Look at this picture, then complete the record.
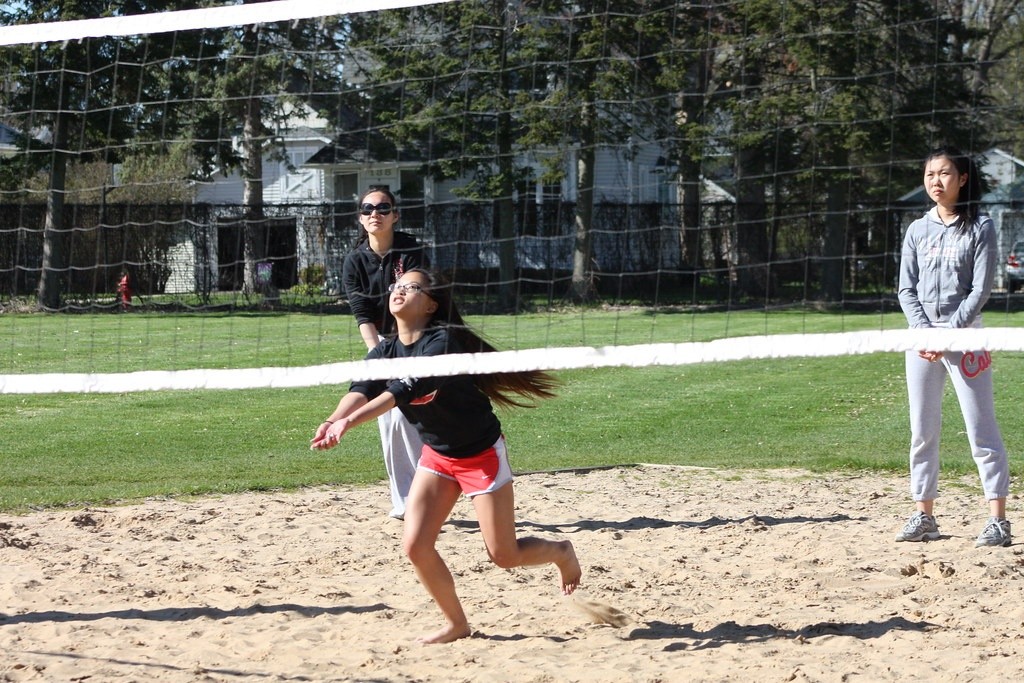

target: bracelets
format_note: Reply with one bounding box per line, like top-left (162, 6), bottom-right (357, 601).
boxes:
top-left (323, 421), bottom-right (334, 424)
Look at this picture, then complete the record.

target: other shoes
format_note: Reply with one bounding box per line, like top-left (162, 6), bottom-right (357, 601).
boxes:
top-left (122, 310), bottom-right (127, 312)
top-left (112, 310), bottom-right (119, 314)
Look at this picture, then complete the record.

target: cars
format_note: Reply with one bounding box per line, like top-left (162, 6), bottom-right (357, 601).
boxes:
top-left (1004, 241), bottom-right (1024, 291)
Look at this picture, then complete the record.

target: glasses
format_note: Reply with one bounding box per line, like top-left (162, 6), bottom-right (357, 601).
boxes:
top-left (388, 284), bottom-right (436, 303)
top-left (359, 202), bottom-right (395, 215)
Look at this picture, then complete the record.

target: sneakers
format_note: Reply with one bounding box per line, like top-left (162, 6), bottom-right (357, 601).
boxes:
top-left (894, 511), bottom-right (941, 542)
top-left (974, 516), bottom-right (1012, 549)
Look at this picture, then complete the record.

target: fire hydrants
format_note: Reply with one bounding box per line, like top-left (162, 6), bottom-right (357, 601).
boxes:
top-left (118, 275), bottom-right (133, 311)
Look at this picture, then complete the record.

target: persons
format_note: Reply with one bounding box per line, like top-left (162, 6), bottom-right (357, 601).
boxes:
top-left (341, 186), bottom-right (431, 521)
top-left (894, 145), bottom-right (1011, 548)
top-left (308, 265), bottom-right (582, 643)
